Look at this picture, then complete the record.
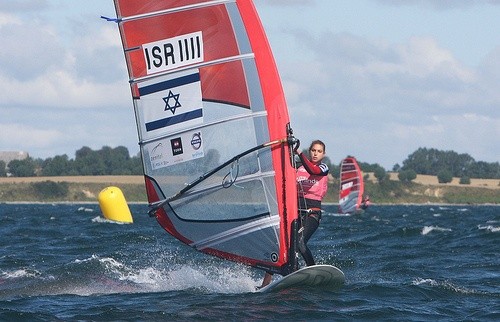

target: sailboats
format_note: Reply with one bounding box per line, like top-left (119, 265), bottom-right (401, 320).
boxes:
top-left (338, 155), bottom-right (366, 218)
top-left (98, 0), bottom-right (344, 295)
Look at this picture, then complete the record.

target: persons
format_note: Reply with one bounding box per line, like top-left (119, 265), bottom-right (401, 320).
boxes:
top-left (360, 194), bottom-right (371, 211)
top-left (287, 140), bottom-right (329, 270)
